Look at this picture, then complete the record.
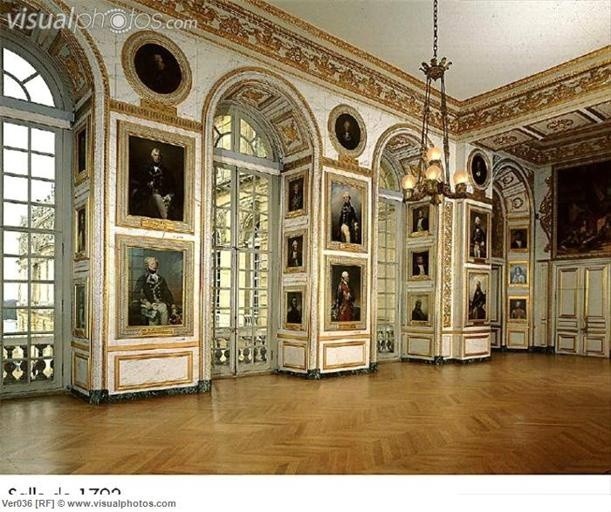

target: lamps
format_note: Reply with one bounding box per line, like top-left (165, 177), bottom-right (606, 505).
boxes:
top-left (399, 1), bottom-right (467, 205)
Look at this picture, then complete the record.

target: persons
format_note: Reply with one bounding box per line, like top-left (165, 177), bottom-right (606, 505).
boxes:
top-left (510, 300), bottom-right (526, 319)
top-left (339, 192), bottom-right (361, 243)
top-left (470, 281), bottom-right (487, 319)
top-left (472, 216), bottom-right (486, 257)
top-left (148, 146), bottom-right (177, 219)
top-left (414, 255), bottom-right (429, 275)
top-left (134, 254), bottom-right (178, 326)
top-left (413, 298), bottom-right (427, 320)
top-left (287, 239), bottom-right (303, 267)
top-left (512, 229), bottom-right (526, 248)
top-left (415, 210), bottom-right (428, 232)
top-left (333, 271), bottom-right (358, 320)
top-left (286, 295), bottom-right (301, 322)
top-left (340, 120), bottom-right (356, 150)
top-left (512, 265), bottom-right (528, 285)
top-left (476, 159), bottom-right (486, 184)
top-left (290, 183), bottom-right (303, 210)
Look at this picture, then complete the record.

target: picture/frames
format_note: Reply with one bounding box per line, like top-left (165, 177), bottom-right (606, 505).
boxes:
top-left (116, 26), bottom-right (195, 340)
top-left (386, 136), bottom-right (529, 323)
top-left (539, 152), bottom-right (610, 259)
top-left (48, 27), bottom-right (93, 108)
top-left (226, 83), bottom-right (370, 331)
top-left (71, 88), bottom-right (100, 351)
